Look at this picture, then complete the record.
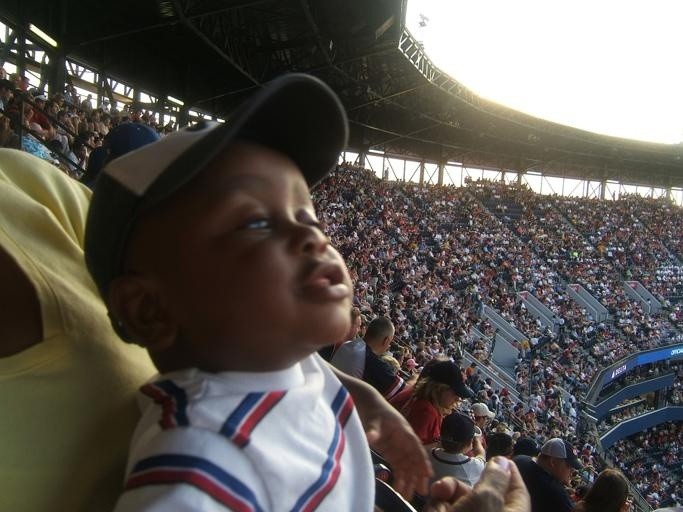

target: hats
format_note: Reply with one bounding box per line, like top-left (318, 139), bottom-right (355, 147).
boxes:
top-left (439, 408), bottom-right (480, 444)
top-left (33, 94), bottom-right (48, 102)
top-left (471, 402), bottom-right (495, 418)
top-left (429, 359), bottom-right (475, 398)
top-left (540, 438), bottom-right (578, 464)
top-left (486, 432), bottom-right (512, 452)
top-left (512, 438), bottom-right (540, 455)
top-left (83, 72), bottom-right (350, 311)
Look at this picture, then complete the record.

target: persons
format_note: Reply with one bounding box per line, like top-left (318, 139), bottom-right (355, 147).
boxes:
top-left (0, 72), bottom-right (683, 511)
